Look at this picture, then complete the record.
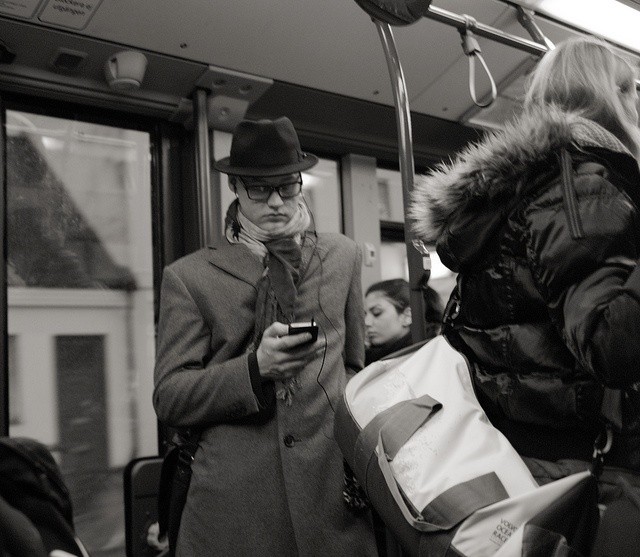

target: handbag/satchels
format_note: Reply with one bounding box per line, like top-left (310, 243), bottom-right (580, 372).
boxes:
top-left (156, 433), bottom-right (194, 556)
top-left (331, 328), bottom-right (600, 557)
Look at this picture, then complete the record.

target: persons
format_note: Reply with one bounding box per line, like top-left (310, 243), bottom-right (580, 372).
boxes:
top-left (362, 279), bottom-right (443, 366)
top-left (405, 35), bottom-right (639, 476)
top-left (153, 114), bottom-right (402, 556)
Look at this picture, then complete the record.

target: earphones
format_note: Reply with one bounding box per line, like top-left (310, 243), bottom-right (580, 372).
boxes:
top-left (229, 178), bottom-right (238, 193)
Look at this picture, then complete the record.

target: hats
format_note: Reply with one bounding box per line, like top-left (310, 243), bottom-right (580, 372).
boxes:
top-left (212, 116), bottom-right (320, 178)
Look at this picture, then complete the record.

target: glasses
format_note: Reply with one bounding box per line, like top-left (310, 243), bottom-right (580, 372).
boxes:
top-left (234, 172), bottom-right (303, 202)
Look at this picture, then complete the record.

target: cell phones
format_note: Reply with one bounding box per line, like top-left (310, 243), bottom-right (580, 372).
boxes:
top-left (288, 321), bottom-right (318, 353)
